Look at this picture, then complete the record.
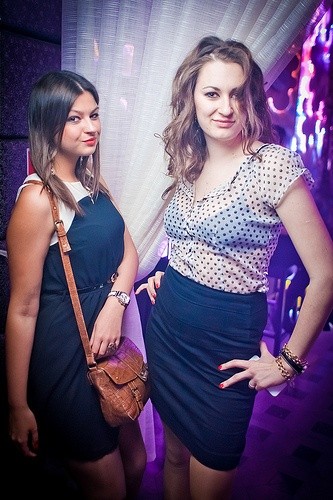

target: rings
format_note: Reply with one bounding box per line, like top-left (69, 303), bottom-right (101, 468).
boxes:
top-left (248, 382), bottom-right (255, 387)
top-left (108, 344), bottom-right (115, 350)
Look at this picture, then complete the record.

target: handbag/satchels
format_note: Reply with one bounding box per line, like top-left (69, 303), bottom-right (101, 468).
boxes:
top-left (86, 336), bottom-right (152, 428)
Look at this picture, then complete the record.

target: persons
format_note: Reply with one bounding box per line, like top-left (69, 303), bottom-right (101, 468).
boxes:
top-left (134, 35), bottom-right (333, 500)
top-left (6, 71), bottom-right (146, 500)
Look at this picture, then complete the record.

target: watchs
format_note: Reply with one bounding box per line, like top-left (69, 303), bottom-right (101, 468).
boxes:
top-left (107, 290), bottom-right (131, 311)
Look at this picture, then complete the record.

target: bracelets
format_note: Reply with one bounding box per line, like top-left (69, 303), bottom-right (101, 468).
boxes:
top-left (275, 345), bottom-right (307, 384)
